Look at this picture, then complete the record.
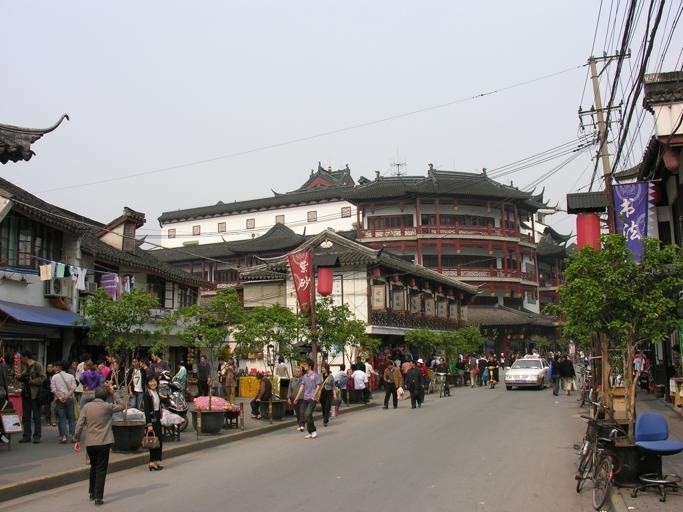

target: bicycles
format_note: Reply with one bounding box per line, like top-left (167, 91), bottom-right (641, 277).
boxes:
top-left (570, 388), bottom-right (625, 508)
top-left (436, 370), bottom-right (452, 397)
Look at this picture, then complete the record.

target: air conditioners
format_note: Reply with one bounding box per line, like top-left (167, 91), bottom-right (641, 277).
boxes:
top-left (45, 279), bottom-right (68, 297)
top-left (81, 282), bottom-right (97, 295)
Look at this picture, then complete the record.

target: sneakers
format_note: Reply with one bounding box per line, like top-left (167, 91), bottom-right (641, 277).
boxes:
top-left (312, 431), bottom-right (318, 437)
top-left (33, 436), bottom-right (40, 443)
top-left (393, 405), bottom-right (397, 409)
top-left (52, 422), bottom-right (57, 426)
top-left (90, 495), bottom-right (95, 500)
top-left (95, 501), bottom-right (104, 505)
top-left (60, 440), bottom-right (67, 443)
top-left (251, 415), bottom-right (257, 417)
top-left (304, 433), bottom-right (311, 438)
top-left (19, 437), bottom-right (30, 443)
top-left (72, 440), bottom-right (78, 443)
top-left (256, 416), bottom-right (262, 419)
top-left (301, 426), bottom-right (305, 430)
top-left (297, 427), bottom-right (301, 431)
top-left (382, 406), bottom-right (387, 408)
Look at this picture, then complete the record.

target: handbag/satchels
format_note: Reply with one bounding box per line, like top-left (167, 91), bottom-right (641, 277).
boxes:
top-left (339, 377), bottom-right (348, 388)
top-left (219, 375), bottom-right (225, 384)
top-left (37, 390), bottom-right (51, 402)
top-left (414, 386), bottom-right (424, 400)
top-left (141, 436), bottom-right (160, 449)
top-left (316, 383), bottom-right (324, 396)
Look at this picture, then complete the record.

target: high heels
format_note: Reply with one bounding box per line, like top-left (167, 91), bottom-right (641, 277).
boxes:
top-left (148, 464), bottom-right (163, 471)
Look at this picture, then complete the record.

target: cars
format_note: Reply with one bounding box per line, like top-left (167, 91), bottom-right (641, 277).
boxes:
top-left (504, 354), bottom-right (554, 390)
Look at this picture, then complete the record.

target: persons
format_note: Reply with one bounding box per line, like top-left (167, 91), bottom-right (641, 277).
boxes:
top-left (172, 355), bottom-right (238, 404)
top-left (382, 357), bottom-right (451, 409)
top-left (548, 351), bottom-right (576, 396)
top-left (0, 353), bottom-right (172, 443)
top-left (250, 356), bottom-right (379, 420)
top-left (294, 358), bottom-right (321, 439)
top-left (457, 353), bottom-right (505, 388)
top-left (71, 382), bottom-right (125, 505)
top-left (143, 373), bottom-right (173, 471)
top-left (286, 366), bottom-right (305, 431)
top-left (672, 344), bottom-right (681, 377)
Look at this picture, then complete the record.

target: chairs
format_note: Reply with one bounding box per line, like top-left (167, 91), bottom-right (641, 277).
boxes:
top-left (630, 413), bottom-right (682, 502)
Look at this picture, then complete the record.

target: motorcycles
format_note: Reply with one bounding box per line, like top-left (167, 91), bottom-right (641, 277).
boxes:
top-left (483, 362), bottom-right (499, 389)
top-left (576, 363), bottom-right (603, 399)
top-left (162, 369), bottom-right (188, 432)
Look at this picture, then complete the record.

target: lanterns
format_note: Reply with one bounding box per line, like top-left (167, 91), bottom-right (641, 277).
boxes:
top-left (423, 280), bottom-right (429, 290)
top-left (448, 289), bottom-right (453, 297)
top-left (437, 285), bottom-right (442, 293)
top-left (459, 293), bottom-right (463, 300)
top-left (409, 278), bottom-right (415, 287)
top-left (392, 273), bottom-right (398, 282)
top-left (576, 212), bottom-right (601, 250)
top-left (372, 268), bottom-right (380, 279)
top-left (317, 268), bottom-right (333, 297)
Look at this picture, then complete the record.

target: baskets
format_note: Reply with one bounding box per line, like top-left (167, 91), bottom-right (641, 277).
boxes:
top-left (587, 422), bottom-right (611, 441)
top-left (436, 376), bottom-right (446, 382)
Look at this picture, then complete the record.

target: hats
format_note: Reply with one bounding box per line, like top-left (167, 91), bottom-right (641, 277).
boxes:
top-left (417, 359), bottom-right (425, 365)
